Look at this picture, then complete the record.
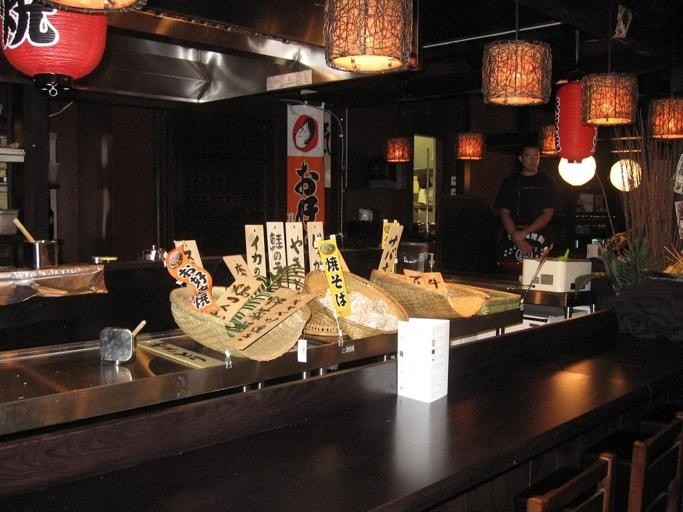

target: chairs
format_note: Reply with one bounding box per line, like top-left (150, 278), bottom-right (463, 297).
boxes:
top-left (512, 400), bottom-right (681, 509)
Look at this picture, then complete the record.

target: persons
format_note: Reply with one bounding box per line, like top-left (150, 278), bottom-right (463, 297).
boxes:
top-left (494, 141), bottom-right (555, 263)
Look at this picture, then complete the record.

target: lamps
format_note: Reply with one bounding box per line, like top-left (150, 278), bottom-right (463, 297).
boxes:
top-left (650, 83), bottom-right (683, 140)
top-left (581, 28), bottom-right (638, 128)
top-left (536, 121), bottom-right (557, 156)
top-left (384, 136), bottom-right (410, 165)
top-left (482, 2), bottom-right (553, 107)
top-left (456, 132), bottom-right (486, 161)
top-left (322, 2), bottom-right (412, 75)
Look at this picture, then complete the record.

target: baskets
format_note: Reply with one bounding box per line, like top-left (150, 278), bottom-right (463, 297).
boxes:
top-left (448, 281), bottom-right (522, 315)
top-left (170, 269), bottom-right (521, 362)
top-left (302, 271), bottom-right (409, 340)
